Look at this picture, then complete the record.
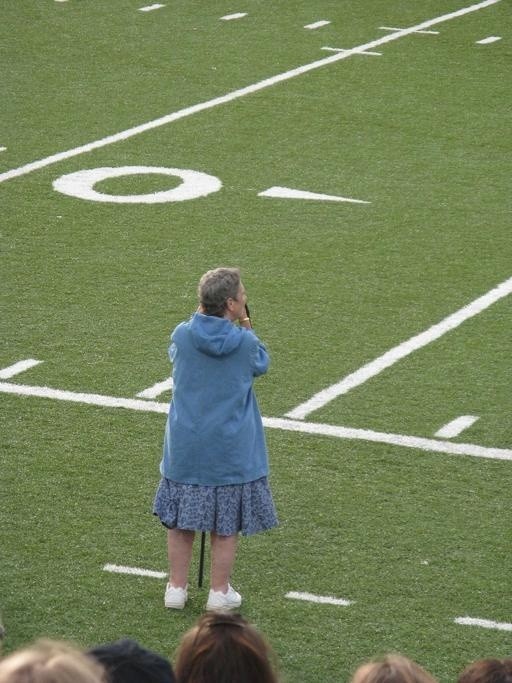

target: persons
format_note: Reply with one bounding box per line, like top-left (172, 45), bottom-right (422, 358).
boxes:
top-left (172, 611), bottom-right (280, 682)
top-left (86, 639), bottom-right (174, 682)
top-left (353, 652), bottom-right (437, 682)
top-left (455, 659), bottom-right (512, 683)
top-left (152, 268), bottom-right (276, 615)
top-left (0, 634), bottom-right (109, 682)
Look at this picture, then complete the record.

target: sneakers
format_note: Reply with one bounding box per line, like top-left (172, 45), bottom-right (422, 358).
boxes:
top-left (163, 583), bottom-right (190, 610)
top-left (207, 583), bottom-right (243, 614)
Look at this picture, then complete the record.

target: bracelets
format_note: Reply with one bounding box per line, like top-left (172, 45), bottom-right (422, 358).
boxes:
top-left (239, 316), bottom-right (250, 324)
